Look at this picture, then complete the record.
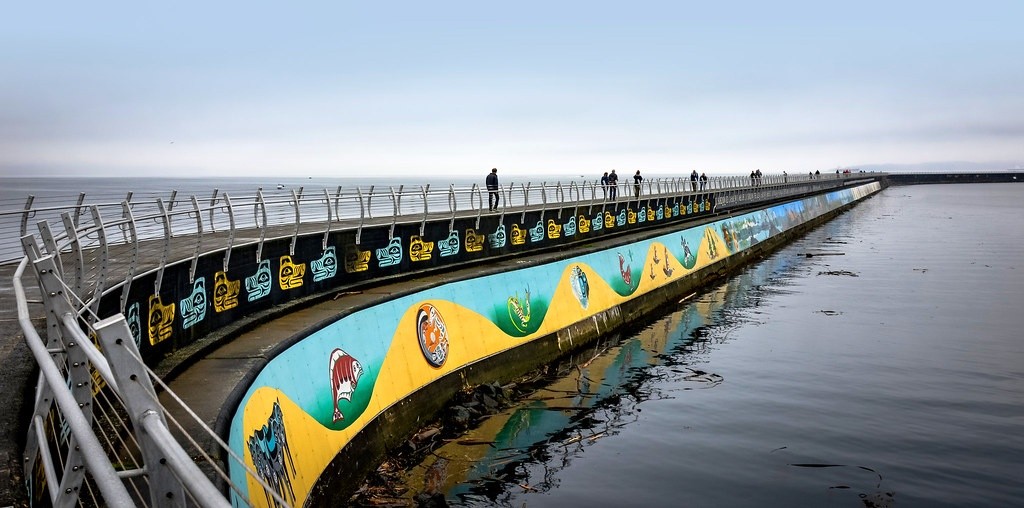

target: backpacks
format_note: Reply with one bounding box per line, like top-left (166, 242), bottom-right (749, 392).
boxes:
top-left (608, 175), bottom-right (617, 186)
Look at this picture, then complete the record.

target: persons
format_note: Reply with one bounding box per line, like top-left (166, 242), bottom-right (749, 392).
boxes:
top-left (633, 170), bottom-right (642, 199)
top-left (783, 171), bottom-right (787, 183)
top-left (750, 169), bottom-right (762, 187)
top-left (836, 170), bottom-right (865, 178)
top-left (601, 172), bottom-right (609, 200)
top-left (609, 170), bottom-right (618, 201)
top-left (809, 172), bottom-right (812, 179)
top-left (691, 170), bottom-right (698, 192)
top-left (700, 173), bottom-right (707, 192)
top-left (815, 170), bottom-right (820, 174)
top-left (486, 168), bottom-right (499, 212)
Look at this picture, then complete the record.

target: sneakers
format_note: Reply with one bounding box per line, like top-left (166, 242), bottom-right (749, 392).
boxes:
top-left (489, 209), bottom-right (497, 212)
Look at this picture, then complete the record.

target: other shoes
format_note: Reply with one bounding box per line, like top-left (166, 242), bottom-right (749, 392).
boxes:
top-left (610, 199), bottom-right (611, 200)
top-left (613, 199), bottom-right (616, 201)
